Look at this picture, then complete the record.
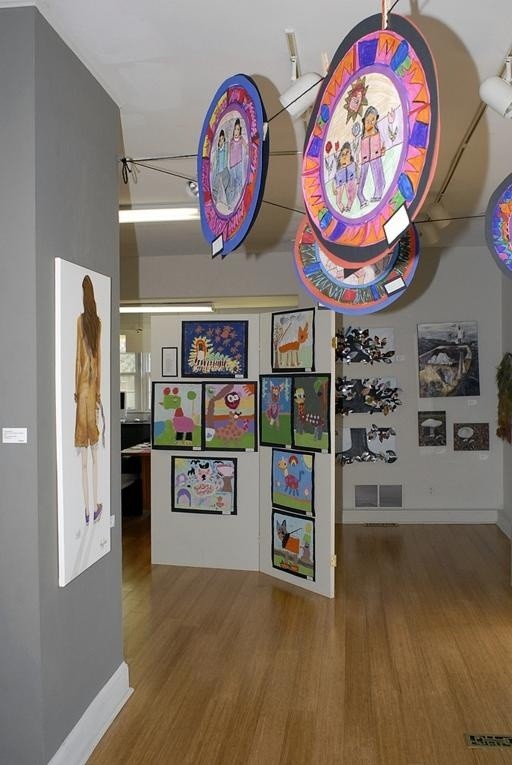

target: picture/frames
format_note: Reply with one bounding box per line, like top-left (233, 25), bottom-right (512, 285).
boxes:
top-left (162, 347), bottom-right (178, 377)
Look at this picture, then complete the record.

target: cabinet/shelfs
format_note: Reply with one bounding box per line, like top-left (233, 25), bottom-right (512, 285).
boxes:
top-left (121, 441), bottom-right (151, 519)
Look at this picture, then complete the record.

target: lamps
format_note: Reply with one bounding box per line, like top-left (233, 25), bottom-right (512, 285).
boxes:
top-left (419, 201), bottom-right (450, 245)
top-left (478, 55), bottom-right (512, 118)
top-left (185, 175), bottom-right (199, 198)
top-left (119, 301), bottom-right (216, 314)
top-left (118, 203), bottom-right (201, 224)
top-left (280, 56), bottom-right (323, 118)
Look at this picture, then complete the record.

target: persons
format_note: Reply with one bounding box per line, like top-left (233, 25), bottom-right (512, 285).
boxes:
top-left (71, 272), bottom-right (109, 531)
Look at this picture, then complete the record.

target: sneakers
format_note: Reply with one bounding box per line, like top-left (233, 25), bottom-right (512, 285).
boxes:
top-left (95, 504), bottom-right (102, 521)
top-left (85, 510), bottom-right (89, 524)
